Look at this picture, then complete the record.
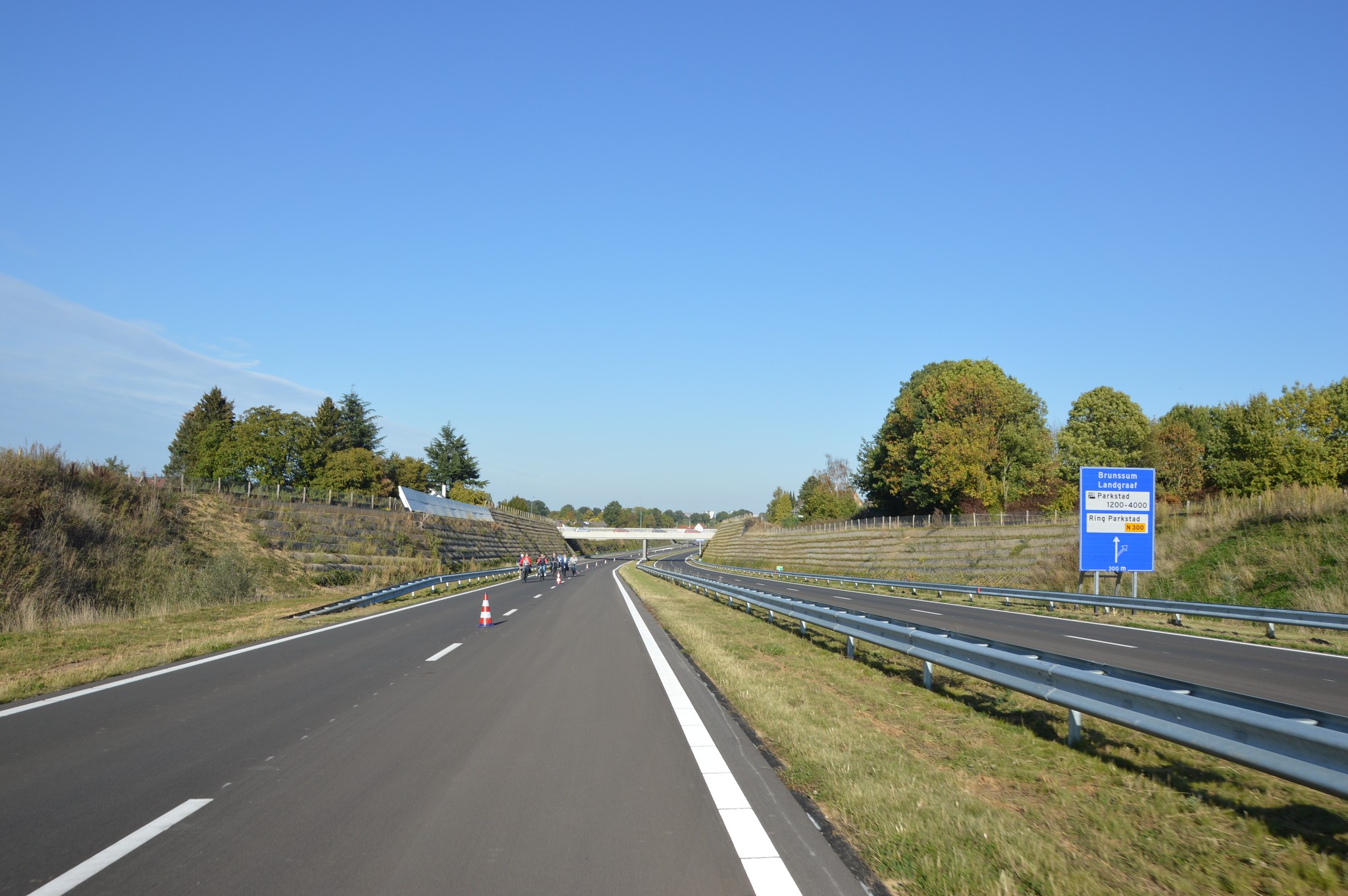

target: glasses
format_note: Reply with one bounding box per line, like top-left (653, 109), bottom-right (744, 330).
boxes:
top-left (541, 556), bottom-right (543, 557)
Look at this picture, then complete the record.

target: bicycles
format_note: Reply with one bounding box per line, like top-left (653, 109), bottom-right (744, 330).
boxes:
top-left (534, 561), bottom-right (577, 581)
top-left (517, 563), bottom-right (530, 583)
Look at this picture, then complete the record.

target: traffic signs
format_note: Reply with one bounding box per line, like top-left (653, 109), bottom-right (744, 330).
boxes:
top-left (1078, 466), bottom-right (1156, 572)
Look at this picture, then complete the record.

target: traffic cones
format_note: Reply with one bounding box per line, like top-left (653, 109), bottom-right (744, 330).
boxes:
top-left (638, 553), bottom-right (640, 556)
top-left (595, 562), bottom-right (598, 567)
top-left (586, 564), bottom-right (589, 570)
top-left (613, 558), bottom-right (616, 562)
top-left (604, 560), bottom-right (607, 564)
top-left (630, 555), bottom-right (632, 558)
top-left (555, 571), bottom-right (563, 585)
top-left (475, 592), bottom-right (496, 628)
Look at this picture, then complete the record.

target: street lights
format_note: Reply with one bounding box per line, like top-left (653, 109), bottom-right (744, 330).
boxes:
top-left (674, 514), bottom-right (678, 528)
top-left (531, 497), bottom-right (535, 518)
top-left (689, 513), bottom-right (693, 529)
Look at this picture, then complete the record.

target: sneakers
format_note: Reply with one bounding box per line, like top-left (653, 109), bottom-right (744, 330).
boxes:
top-left (519, 576), bottom-right (521, 578)
top-left (536, 573), bottom-right (538, 577)
top-left (540, 573), bottom-right (542, 577)
top-left (538, 573), bottom-right (540, 577)
top-left (544, 573), bottom-right (546, 576)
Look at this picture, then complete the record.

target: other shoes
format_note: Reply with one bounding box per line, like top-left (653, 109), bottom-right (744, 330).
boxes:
top-left (526, 573), bottom-right (528, 577)
top-left (554, 571), bottom-right (556, 573)
top-left (551, 571), bottom-right (553, 573)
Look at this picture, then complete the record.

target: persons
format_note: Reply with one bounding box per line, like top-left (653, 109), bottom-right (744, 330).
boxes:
top-left (517, 553), bottom-right (579, 578)
